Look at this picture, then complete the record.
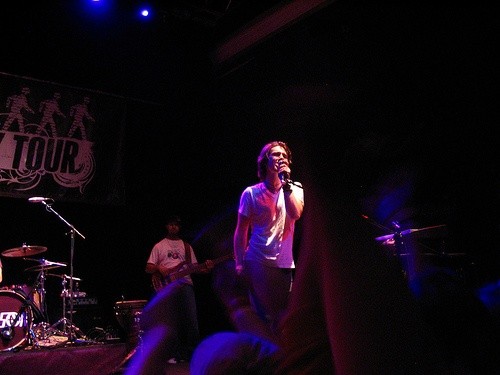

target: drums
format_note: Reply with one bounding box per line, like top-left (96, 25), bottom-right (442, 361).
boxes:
top-left (0, 289), bottom-right (33, 352)
top-left (115, 300), bottom-right (148, 345)
top-left (1, 286), bottom-right (41, 318)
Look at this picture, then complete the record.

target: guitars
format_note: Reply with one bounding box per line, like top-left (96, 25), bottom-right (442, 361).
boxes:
top-left (152, 253), bottom-right (233, 293)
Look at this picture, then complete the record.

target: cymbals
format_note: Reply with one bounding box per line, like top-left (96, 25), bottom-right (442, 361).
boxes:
top-left (1, 245), bottom-right (47, 257)
top-left (25, 262), bottom-right (68, 272)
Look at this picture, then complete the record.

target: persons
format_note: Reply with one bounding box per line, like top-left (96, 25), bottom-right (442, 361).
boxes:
top-left (190, 268), bottom-right (500, 375)
top-left (234, 140), bottom-right (304, 336)
top-left (146, 212), bottom-right (199, 363)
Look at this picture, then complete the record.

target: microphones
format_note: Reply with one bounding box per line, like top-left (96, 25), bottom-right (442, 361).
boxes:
top-left (277, 160), bottom-right (286, 182)
top-left (28, 197), bottom-right (51, 203)
top-left (2, 331), bottom-right (11, 339)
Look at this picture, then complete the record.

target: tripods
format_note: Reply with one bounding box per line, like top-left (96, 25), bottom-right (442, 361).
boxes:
top-left (46, 277), bottom-right (87, 341)
